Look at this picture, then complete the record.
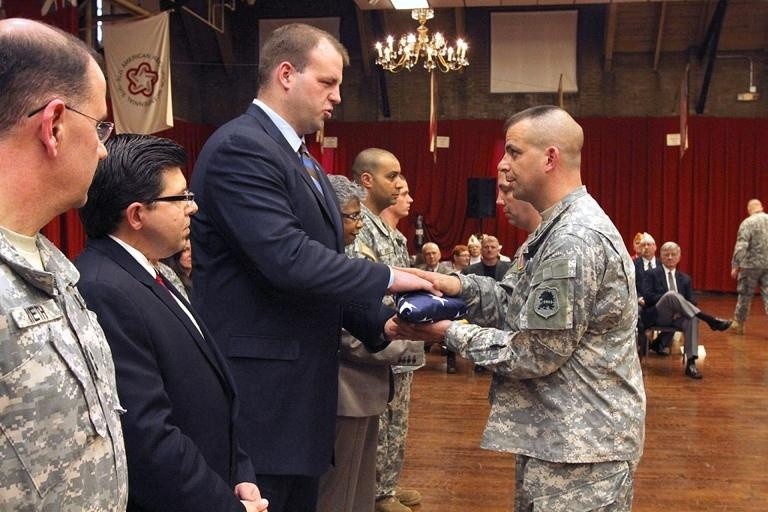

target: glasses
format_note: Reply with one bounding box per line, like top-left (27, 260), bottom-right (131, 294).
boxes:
top-left (455, 253), bottom-right (471, 258)
top-left (28, 100), bottom-right (115, 145)
top-left (147, 190), bottom-right (196, 205)
top-left (341, 212), bottom-right (369, 225)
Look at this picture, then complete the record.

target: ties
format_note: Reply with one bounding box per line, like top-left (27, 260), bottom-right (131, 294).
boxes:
top-left (152, 267), bottom-right (171, 295)
top-left (647, 260), bottom-right (654, 272)
top-left (668, 270), bottom-right (678, 294)
top-left (296, 142), bottom-right (326, 202)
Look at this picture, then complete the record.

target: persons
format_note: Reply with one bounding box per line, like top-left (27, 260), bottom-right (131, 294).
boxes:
top-left (633, 235), bottom-right (675, 355)
top-left (464, 236), bottom-right (511, 280)
top-left (479, 233), bottom-right (490, 242)
top-left (321, 168), bottom-right (400, 512)
top-left (627, 231), bottom-right (644, 261)
top-left (441, 245), bottom-right (468, 276)
top-left (390, 106), bottom-right (647, 512)
top-left (1, 16), bottom-right (129, 512)
top-left (72, 132), bottom-right (270, 512)
top-left (456, 155), bottom-right (543, 512)
top-left (190, 22), bottom-right (441, 512)
top-left (379, 174), bottom-right (429, 512)
top-left (728, 199), bottom-right (768, 331)
top-left (639, 240), bottom-right (732, 381)
top-left (466, 235), bottom-right (482, 266)
top-left (348, 141), bottom-right (401, 512)
top-left (411, 240), bottom-right (452, 275)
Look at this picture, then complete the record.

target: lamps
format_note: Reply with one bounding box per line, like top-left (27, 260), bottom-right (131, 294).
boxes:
top-left (374, 9), bottom-right (469, 73)
top-left (390, 0), bottom-right (430, 9)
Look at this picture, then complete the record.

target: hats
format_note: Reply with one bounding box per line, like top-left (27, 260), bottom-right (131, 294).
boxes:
top-left (468, 235), bottom-right (480, 247)
top-left (640, 233), bottom-right (655, 244)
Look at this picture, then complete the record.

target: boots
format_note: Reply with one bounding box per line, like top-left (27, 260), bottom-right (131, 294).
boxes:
top-left (392, 484), bottom-right (423, 507)
top-left (375, 493), bottom-right (409, 512)
top-left (728, 318), bottom-right (746, 337)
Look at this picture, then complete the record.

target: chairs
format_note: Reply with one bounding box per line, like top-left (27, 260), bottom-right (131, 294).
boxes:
top-left (645, 325), bottom-right (686, 369)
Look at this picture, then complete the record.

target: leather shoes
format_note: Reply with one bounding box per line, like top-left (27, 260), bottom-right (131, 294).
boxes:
top-left (653, 338), bottom-right (670, 356)
top-left (684, 362), bottom-right (704, 382)
top-left (709, 318), bottom-right (735, 333)
